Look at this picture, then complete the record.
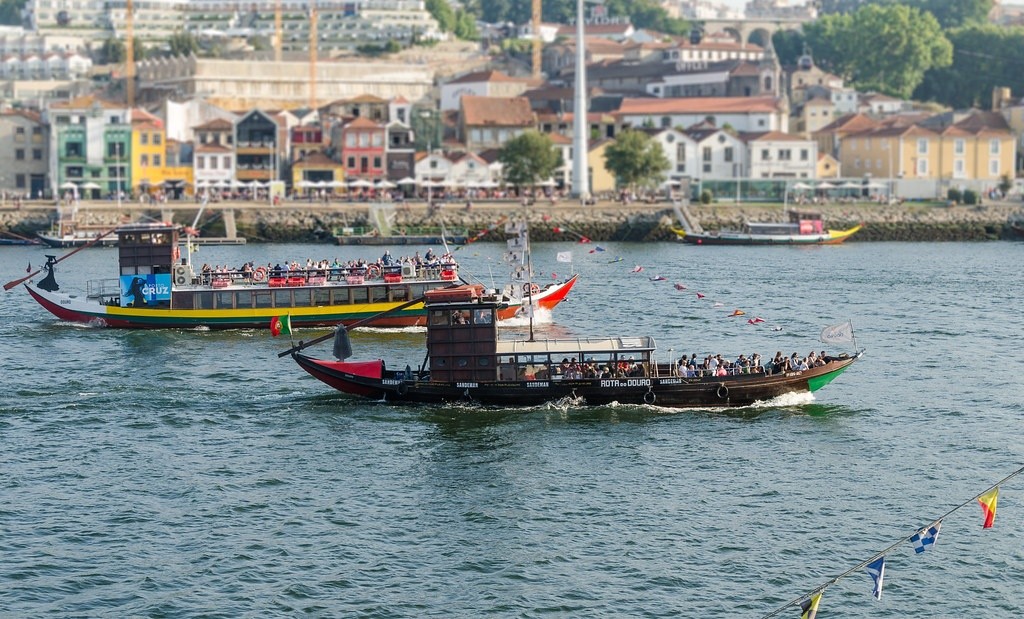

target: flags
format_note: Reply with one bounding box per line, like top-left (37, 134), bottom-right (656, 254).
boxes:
top-left (440, 211), bottom-right (783, 333)
top-left (977, 487), bottom-right (998, 528)
top-left (908, 521), bottom-right (943, 553)
top-left (864, 557), bottom-right (886, 600)
top-left (798, 591), bottom-right (822, 619)
top-left (821, 324), bottom-right (853, 343)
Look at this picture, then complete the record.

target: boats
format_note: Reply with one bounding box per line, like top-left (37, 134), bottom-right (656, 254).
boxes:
top-left (35, 192), bottom-right (248, 248)
top-left (23, 180), bottom-right (580, 330)
top-left (669, 191), bottom-right (863, 246)
top-left (280, 227), bottom-right (868, 407)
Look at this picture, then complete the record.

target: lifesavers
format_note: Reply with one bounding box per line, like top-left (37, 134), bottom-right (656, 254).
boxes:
top-left (715, 386), bottom-right (730, 399)
top-left (523, 282), bottom-right (530, 293)
top-left (252, 267), bottom-right (267, 283)
top-left (397, 382), bottom-right (409, 395)
top-left (531, 284), bottom-right (541, 294)
top-left (643, 391), bottom-right (658, 405)
top-left (365, 263), bottom-right (381, 279)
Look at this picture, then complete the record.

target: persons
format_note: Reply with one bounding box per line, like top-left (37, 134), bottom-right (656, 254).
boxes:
top-left (36, 257), bottom-right (60, 292)
top-left (477, 310), bottom-right (486, 324)
top-left (406, 350), bottom-right (831, 379)
top-left (201, 247), bottom-right (455, 283)
top-left (124, 277), bottom-right (146, 307)
top-left (0, 187), bottom-right (904, 238)
top-left (452, 311), bottom-right (468, 325)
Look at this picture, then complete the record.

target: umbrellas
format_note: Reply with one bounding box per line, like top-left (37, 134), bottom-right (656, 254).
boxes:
top-left (53, 177), bottom-right (886, 190)
